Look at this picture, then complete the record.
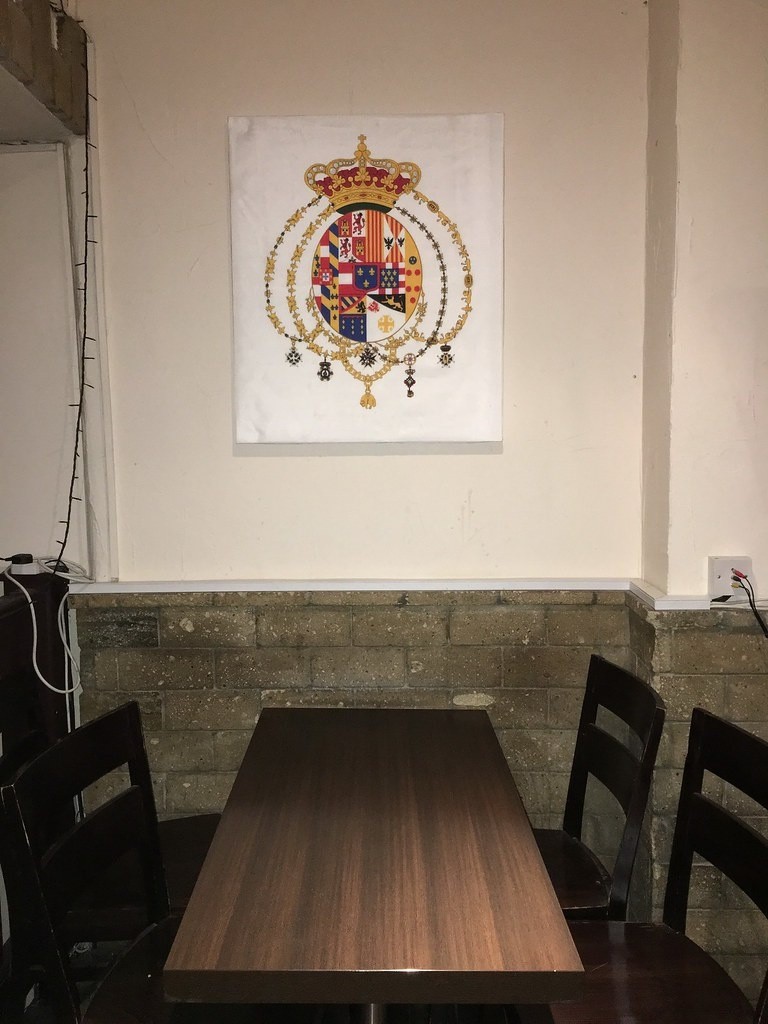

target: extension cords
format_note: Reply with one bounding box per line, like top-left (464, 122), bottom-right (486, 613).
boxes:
top-left (11, 561), bottom-right (47, 574)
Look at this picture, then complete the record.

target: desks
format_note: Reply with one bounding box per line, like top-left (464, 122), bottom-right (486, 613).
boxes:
top-left (161, 708), bottom-right (586, 1024)
top-left (0, 569), bottom-right (75, 975)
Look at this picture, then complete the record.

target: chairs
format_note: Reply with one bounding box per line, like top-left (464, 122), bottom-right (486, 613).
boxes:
top-left (507, 705), bottom-right (768, 1024)
top-left (0, 700), bottom-right (351, 1024)
top-left (532, 652), bottom-right (668, 920)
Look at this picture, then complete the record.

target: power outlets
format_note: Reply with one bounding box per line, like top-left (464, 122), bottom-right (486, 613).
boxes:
top-left (707, 556), bottom-right (753, 602)
